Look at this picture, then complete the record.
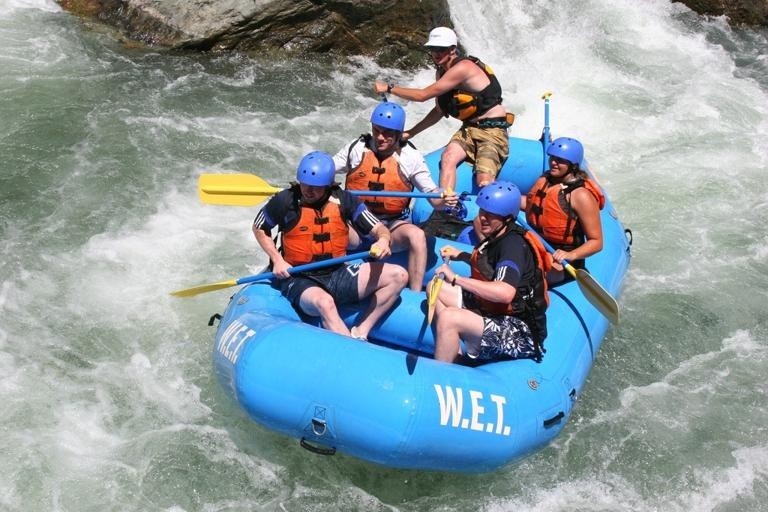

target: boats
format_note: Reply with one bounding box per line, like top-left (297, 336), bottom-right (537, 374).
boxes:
top-left (204, 130), bottom-right (638, 476)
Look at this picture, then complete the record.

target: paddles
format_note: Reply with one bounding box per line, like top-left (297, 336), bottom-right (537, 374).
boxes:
top-left (428, 255), bottom-right (450, 325)
top-left (515, 216), bottom-right (619, 327)
top-left (199, 173), bottom-right (453, 206)
top-left (172, 248), bottom-right (379, 299)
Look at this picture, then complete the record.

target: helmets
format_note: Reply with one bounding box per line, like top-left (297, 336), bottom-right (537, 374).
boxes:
top-left (296, 151), bottom-right (335, 187)
top-left (546, 137), bottom-right (584, 167)
top-left (474, 181), bottom-right (521, 220)
top-left (370, 102), bottom-right (405, 132)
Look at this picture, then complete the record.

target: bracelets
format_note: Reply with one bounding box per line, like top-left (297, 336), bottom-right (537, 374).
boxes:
top-left (452, 274), bottom-right (459, 286)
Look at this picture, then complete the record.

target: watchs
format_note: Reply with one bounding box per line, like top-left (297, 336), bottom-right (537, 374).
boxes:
top-left (387, 83), bottom-right (394, 93)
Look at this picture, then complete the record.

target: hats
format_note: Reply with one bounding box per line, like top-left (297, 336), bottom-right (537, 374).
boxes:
top-left (423, 26), bottom-right (457, 48)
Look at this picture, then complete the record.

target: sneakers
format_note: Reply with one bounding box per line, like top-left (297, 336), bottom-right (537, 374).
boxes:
top-left (349, 326), bottom-right (368, 342)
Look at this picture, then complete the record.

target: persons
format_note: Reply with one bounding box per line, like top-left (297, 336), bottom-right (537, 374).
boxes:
top-left (332, 102), bottom-right (459, 291)
top-left (474, 137), bottom-right (605, 290)
top-left (374, 27), bottom-right (509, 194)
top-left (426, 181), bottom-right (550, 364)
top-left (252, 150), bottom-right (408, 341)
